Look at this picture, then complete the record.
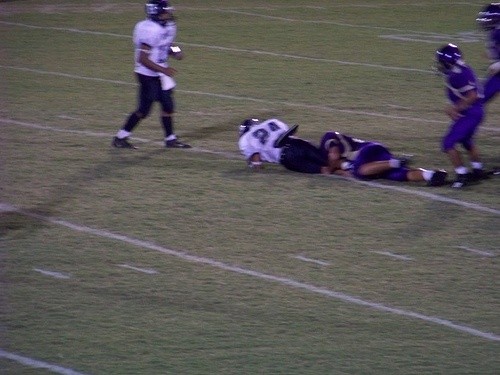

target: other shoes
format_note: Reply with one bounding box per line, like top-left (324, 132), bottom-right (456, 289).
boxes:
top-left (490, 167), bottom-right (500, 175)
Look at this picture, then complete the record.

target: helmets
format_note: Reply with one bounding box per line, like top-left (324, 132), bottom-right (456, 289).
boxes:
top-left (144, 0), bottom-right (176, 21)
top-left (435, 44), bottom-right (462, 74)
top-left (239, 118), bottom-right (264, 137)
top-left (476, 3), bottom-right (500, 30)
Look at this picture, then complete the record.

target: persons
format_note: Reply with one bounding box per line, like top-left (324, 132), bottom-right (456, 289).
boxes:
top-left (321, 131), bottom-right (447, 183)
top-left (435, 43), bottom-right (484, 189)
top-left (476, 3), bottom-right (500, 100)
top-left (239, 118), bottom-right (351, 177)
top-left (112, 0), bottom-right (192, 149)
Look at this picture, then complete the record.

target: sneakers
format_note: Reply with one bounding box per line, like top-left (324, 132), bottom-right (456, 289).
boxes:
top-left (469, 169), bottom-right (487, 179)
top-left (430, 169), bottom-right (447, 186)
top-left (113, 137), bottom-right (137, 149)
top-left (452, 174), bottom-right (470, 188)
top-left (167, 141), bottom-right (192, 148)
top-left (394, 158), bottom-right (410, 166)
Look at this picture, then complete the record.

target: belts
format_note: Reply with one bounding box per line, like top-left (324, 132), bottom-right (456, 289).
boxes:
top-left (280, 142), bottom-right (291, 164)
